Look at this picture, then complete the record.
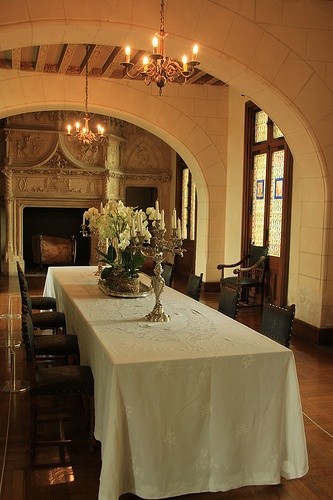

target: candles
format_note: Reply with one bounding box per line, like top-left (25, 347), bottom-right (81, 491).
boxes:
top-left (155, 199), bottom-right (160, 220)
top-left (83, 212), bottom-right (85, 225)
top-left (131, 218), bottom-right (136, 236)
top-left (177, 217), bottom-right (182, 239)
top-left (160, 209), bottom-right (165, 228)
top-left (137, 209), bottom-right (143, 231)
top-left (172, 208), bottom-right (176, 228)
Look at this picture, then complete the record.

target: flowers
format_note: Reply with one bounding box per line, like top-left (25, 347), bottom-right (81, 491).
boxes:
top-left (86, 200), bottom-right (166, 279)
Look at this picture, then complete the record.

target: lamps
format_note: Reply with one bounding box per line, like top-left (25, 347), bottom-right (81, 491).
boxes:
top-left (119, 0), bottom-right (201, 97)
top-left (66, 46), bottom-right (106, 151)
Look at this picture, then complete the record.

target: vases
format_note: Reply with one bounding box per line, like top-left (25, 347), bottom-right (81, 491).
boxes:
top-left (98, 267), bottom-right (151, 299)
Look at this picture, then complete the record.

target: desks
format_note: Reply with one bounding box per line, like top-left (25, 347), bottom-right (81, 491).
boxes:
top-left (40, 266), bottom-right (309, 500)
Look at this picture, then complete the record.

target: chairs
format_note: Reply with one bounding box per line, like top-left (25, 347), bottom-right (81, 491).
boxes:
top-left (216, 279), bottom-right (241, 319)
top-left (14, 261), bottom-right (102, 500)
top-left (217, 242), bottom-right (269, 314)
top-left (260, 301), bottom-right (296, 350)
top-left (161, 261), bottom-right (176, 287)
top-left (186, 270), bottom-right (205, 302)
